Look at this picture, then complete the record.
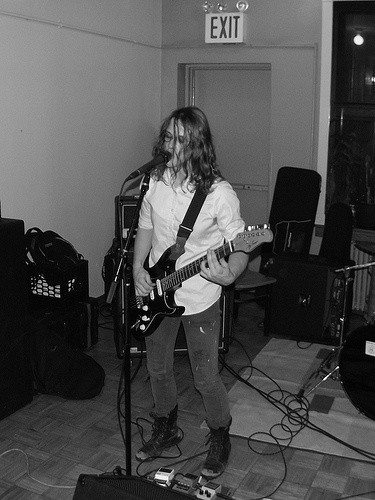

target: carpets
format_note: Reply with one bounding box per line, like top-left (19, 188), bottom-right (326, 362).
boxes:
top-left (201, 338), bottom-right (375, 463)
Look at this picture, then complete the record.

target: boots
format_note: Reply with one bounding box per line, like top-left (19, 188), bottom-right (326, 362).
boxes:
top-left (201, 422), bottom-right (232, 479)
top-left (136, 404), bottom-right (179, 463)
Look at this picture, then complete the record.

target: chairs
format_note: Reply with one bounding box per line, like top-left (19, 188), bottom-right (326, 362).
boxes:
top-left (223, 268), bottom-right (276, 344)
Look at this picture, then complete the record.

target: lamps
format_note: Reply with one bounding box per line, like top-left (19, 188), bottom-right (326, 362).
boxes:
top-left (353, 29), bottom-right (364, 45)
top-left (200, 0), bottom-right (249, 45)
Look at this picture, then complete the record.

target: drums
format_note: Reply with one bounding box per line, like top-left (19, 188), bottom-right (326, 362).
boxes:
top-left (338, 322), bottom-right (375, 422)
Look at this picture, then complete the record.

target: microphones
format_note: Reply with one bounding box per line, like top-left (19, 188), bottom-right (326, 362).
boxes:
top-left (124, 151), bottom-right (172, 183)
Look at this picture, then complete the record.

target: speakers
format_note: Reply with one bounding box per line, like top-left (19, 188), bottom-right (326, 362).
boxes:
top-left (0, 217), bottom-right (35, 421)
top-left (115, 247), bottom-right (232, 358)
top-left (258, 166), bottom-right (356, 343)
top-left (73, 474), bottom-right (201, 500)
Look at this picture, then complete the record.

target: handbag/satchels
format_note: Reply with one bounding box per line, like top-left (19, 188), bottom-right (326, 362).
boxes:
top-left (38, 349), bottom-right (104, 400)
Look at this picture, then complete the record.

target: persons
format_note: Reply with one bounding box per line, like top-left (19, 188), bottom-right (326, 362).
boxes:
top-left (134, 107), bottom-right (251, 481)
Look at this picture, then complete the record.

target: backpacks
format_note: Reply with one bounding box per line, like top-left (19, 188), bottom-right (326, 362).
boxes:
top-left (24, 227), bottom-right (83, 287)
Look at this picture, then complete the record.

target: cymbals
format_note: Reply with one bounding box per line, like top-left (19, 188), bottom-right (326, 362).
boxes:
top-left (353, 239), bottom-right (375, 254)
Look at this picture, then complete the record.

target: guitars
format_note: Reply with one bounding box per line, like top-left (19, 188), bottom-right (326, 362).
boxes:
top-left (125, 219), bottom-right (275, 340)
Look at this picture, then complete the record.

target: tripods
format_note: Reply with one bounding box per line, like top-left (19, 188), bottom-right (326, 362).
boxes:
top-left (297, 261), bottom-right (375, 399)
